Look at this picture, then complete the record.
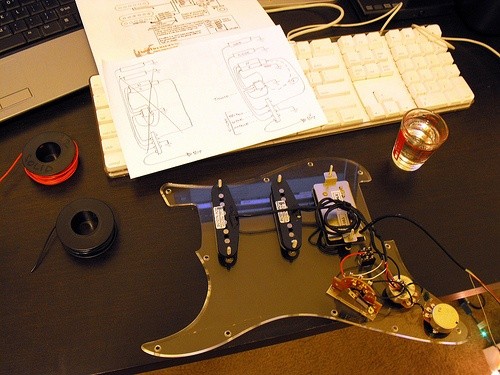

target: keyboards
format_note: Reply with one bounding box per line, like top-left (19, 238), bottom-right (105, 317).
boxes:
top-left (89, 23), bottom-right (476, 178)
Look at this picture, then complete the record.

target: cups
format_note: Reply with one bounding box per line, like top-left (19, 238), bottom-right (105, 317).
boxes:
top-left (392, 108), bottom-right (448, 170)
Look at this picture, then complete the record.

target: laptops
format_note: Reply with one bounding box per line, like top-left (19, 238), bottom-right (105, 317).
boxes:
top-left (1, 0), bottom-right (99, 123)
